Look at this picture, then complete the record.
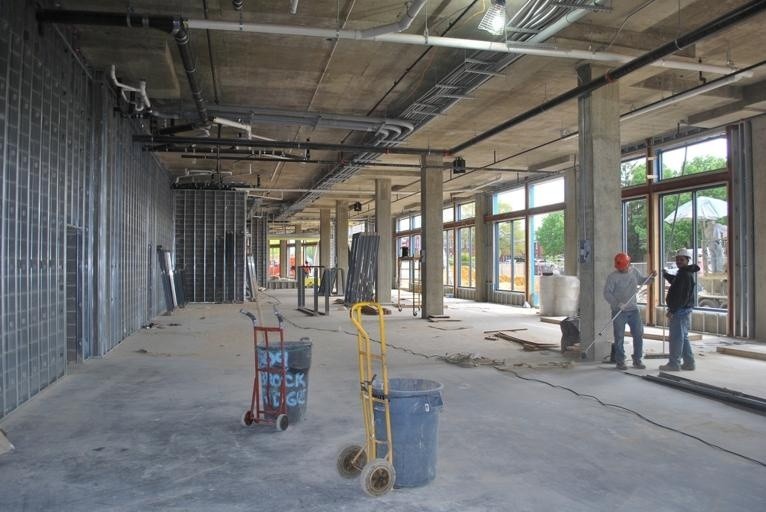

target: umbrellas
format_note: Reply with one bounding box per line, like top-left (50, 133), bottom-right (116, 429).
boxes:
top-left (664, 196), bottom-right (728, 272)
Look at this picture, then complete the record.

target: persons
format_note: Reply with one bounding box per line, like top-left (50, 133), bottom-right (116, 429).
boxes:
top-left (304, 261), bottom-right (311, 279)
top-left (706, 220), bottom-right (723, 274)
top-left (604, 253), bottom-right (657, 370)
top-left (659, 247), bottom-right (700, 370)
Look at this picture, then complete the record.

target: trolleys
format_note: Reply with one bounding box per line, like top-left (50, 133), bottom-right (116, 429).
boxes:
top-left (336, 302), bottom-right (396, 497)
top-left (240, 308), bottom-right (289, 432)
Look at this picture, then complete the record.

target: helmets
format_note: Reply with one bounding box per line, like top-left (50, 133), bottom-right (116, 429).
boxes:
top-left (615, 254), bottom-right (631, 268)
top-left (675, 248), bottom-right (692, 260)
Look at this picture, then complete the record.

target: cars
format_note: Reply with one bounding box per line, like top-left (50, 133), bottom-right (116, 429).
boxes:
top-left (535, 258), bottom-right (558, 275)
top-left (664, 260), bottom-right (713, 299)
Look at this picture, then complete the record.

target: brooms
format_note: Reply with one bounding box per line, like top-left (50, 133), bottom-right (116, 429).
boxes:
top-left (645, 274), bottom-right (672, 359)
top-left (563, 272), bottom-right (654, 363)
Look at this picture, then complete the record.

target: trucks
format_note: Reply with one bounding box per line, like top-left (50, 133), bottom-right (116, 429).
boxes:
top-left (269, 255), bottom-right (295, 279)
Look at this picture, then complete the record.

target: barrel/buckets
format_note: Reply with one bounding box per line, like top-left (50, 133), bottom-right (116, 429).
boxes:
top-left (539, 272), bottom-right (581, 317)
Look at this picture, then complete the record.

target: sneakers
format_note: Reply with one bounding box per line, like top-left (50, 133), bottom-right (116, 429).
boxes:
top-left (616, 360), bottom-right (628, 370)
top-left (659, 362), bottom-right (695, 371)
top-left (633, 360), bottom-right (646, 369)
